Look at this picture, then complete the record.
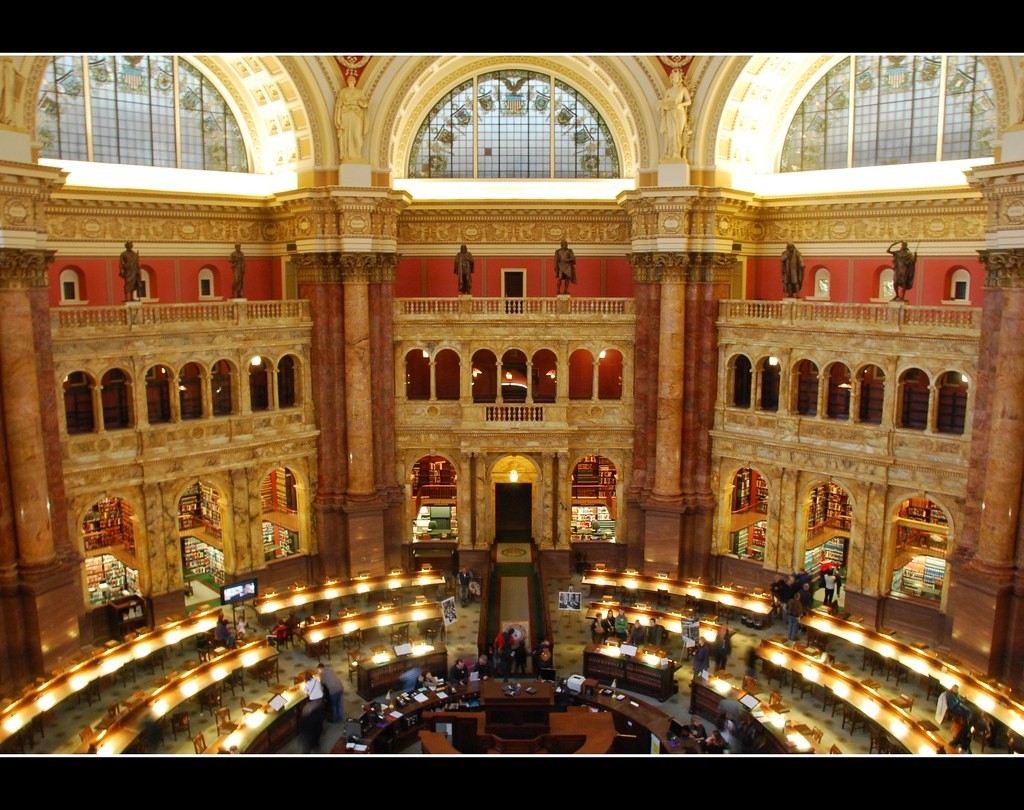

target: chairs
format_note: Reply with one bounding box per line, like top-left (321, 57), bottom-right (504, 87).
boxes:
top-left (443, 576), bottom-right (459, 602)
top-left (596, 563), bottom-right (1024, 754)
top-left (253, 564), bottom-right (438, 683)
top-left (0, 604), bottom-right (317, 754)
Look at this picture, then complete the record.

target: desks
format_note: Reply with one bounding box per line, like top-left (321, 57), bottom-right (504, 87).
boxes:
top-left (291, 601), bottom-right (443, 658)
top-left (479, 680), bottom-right (555, 725)
top-left (0, 608), bottom-right (229, 753)
top-left (417, 730), bottom-right (462, 754)
top-left (585, 601), bottom-right (736, 660)
top-left (356, 643), bottom-right (448, 701)
top-left (555, 683), bottom-right (709, 754)
top-left (756, 640), bottom-right (959, 754)
top-left (688, 675), bottom-right (827, 755)
top-left (582, 568), bottom-right (783, 630)
top-left (583, 643), bottom-right (683, 703)
top-left (549, 712), bottom-right (616, 753)
top-left (202, 673), bottom-right (330, 754)
top-left (72, 638), bottom-right (280, 754)
top-left (254, 569), bottom-right (445, 628)
top-left (799, 609), bottom-right (1024, 737)
top-left (330, 681), bottom-right (480, 756)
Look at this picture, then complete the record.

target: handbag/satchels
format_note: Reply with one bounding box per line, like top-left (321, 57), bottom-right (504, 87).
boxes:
top-left (818, 580), bottom-right (826, 588)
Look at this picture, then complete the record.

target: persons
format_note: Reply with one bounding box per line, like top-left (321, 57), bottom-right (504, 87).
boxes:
top-left (887, 240), bottom-right (917, 301)
top-left (780, 242), bottom-right (805, 298)
top-left (266, 611), bottom-right (294, 646)
top-left (414, 671), bottom-right (438, 690)
top-left (591, 607), bottom-right (663, 650)
top-left (235, 617), bottom-right (248, 641)
top-left (492, 628), bottom-right (535, 683)
top-left (944, 685), bottom-right (975, 754)
top-left (770, 567), bottom-right (837, 641)
top-left (118, 240), bottom-right (141, 301)
top-left (554, 240), bottom-right (575, 295)
top-left (683, 714), bottom-right (727, 755)
top-left (457, 567), bottom-right (473, 608)
top-left (213, 614), bottom-right (236, 648)
top-left (449, 658), bottom-right (470, 685)
top-left (303, 664), bottom-right (344, 726)
top-left (692, 638), bottom-right (710, 678)
top-left (228, 243), bottom-right (246, 298)
top-left (453, 244), bottom-right (475, 294)
top-left (714, 625), bottom-right (733, 675)
top-left (471, 655), bottom-right (492, 681)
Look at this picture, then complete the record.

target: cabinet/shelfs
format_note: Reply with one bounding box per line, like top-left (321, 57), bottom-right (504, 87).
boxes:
top-left (109, 595), bottom-right (150, 640)
top-left (732, 468), bottom-right (768, 514)
top-left (572, 455), bottom-right (617, 498)
top-left (177, 479), bottom-right (222, 541)
top-left (81, 495), bottom-right (135, 555)
top-left (260, 467), bottom-right (297, 514)
top-left (411, 455), bottom-right (457, 498)
top-left (85, 556), bottom-right (140, 594)
top-left (892, 497), bottom-right (948, 556)
top-left (806, 481), bottom-right (853, 540)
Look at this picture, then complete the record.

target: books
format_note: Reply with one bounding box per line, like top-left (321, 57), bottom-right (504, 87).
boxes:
top-left (78, 470), bottom-right (294, 605)
top-left (737, 468), bottom-right (847, 568)
top-left (572, 456), bottom-right (615, 497)
top-left (412, 463), bottom-right (456, 485)
top-left (892, 501), bottom-right (948, 600)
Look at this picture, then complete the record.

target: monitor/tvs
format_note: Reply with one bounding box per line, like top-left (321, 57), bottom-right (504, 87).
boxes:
top-left (220, 577), bottom-right (257, 605)
